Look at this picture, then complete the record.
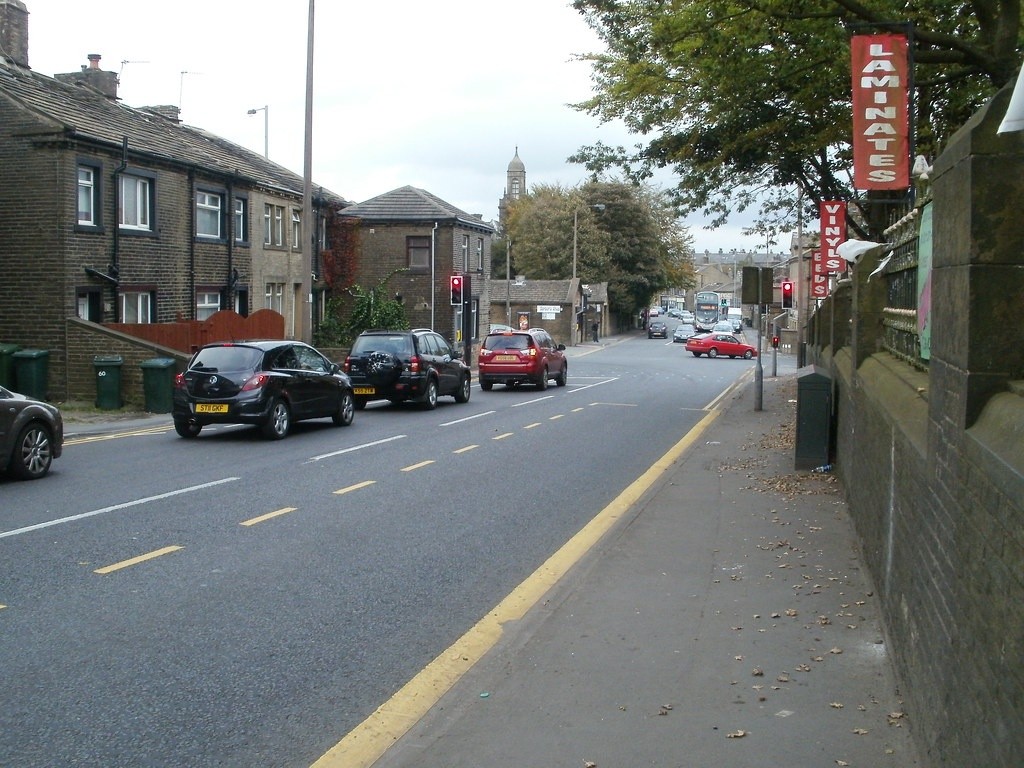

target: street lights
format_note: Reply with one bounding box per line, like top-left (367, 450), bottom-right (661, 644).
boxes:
top-left (572, 203), bottom-right (604, 277)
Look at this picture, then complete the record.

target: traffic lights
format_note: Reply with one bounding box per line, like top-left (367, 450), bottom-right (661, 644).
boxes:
top-left (450, 275), bottom-right (463, 306)
top-left (772, 336), bottom-right (779, 347)
top-left (782, 281), bottom-right (792, 309)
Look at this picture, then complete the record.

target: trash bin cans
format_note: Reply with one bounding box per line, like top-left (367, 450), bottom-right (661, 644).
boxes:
top-left (12, 349), bottom-right (53, 402)
top-left (92, 355), bottom-right (125, 409)
top-left (0, 343), bottom-right (22, 391)
top-left (793, 364), bottom-right (836, 471)
top-left (139, 358), bottom-right (177, 413)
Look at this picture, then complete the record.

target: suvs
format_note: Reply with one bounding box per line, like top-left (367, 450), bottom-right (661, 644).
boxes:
top-left (343, 327), bottom-right (473, 410)
top-left (477, 327), bottom-right (567, 393)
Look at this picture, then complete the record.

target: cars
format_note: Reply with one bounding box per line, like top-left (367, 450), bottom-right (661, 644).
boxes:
top-left (648, 321), bottom-right (668, 339)
top-left (171, 337), bottom-right (354, 440)
top-left (648, 305), bottom-right (694, 323)
top-left (710, 307), bottom-right (744, 339)
top-left (685, 332), bottom-right (757, 360)
top-left (1, 380), bottom-right (65, 478)
top-left (672, 324), bottom-right (699, 342)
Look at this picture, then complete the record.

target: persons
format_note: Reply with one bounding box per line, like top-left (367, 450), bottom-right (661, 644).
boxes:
top-left (591, 321), bottom-right (599, 342)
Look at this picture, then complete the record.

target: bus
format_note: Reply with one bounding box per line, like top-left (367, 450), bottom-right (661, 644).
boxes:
top-left (694, 291), bottom-right (721, 332)
